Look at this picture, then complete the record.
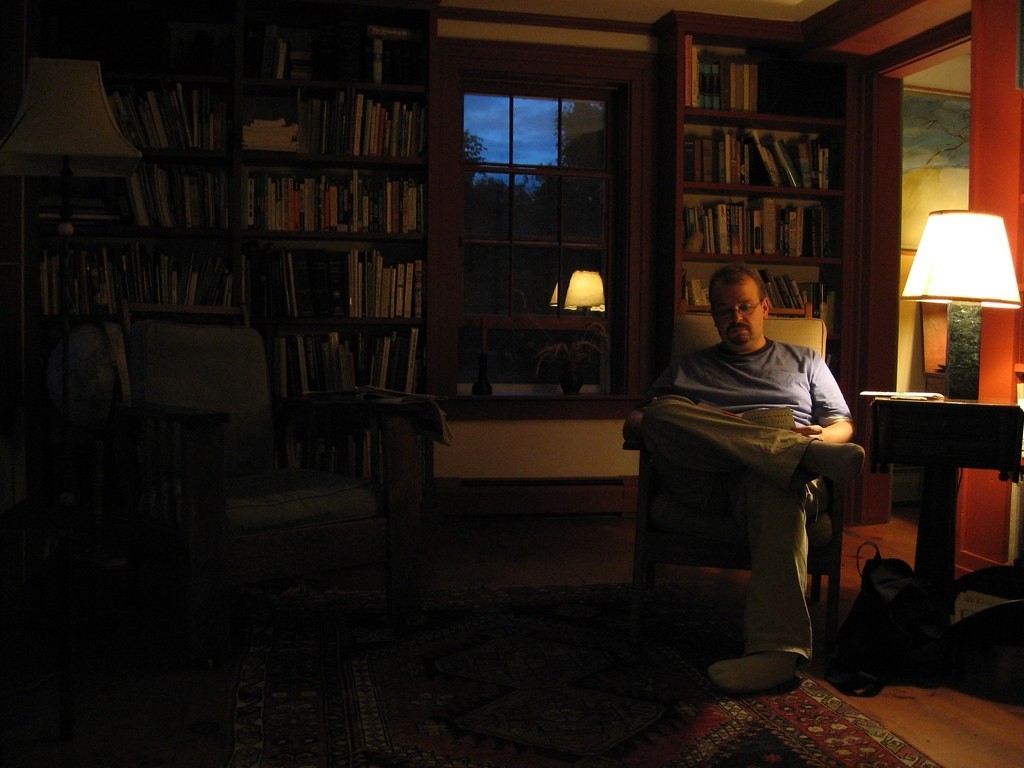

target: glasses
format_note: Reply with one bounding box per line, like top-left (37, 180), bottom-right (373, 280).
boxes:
top-left (712, 299), bottom-right (763, 320)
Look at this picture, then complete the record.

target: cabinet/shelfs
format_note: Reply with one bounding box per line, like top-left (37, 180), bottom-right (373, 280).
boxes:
top-left (40, 0), bottom-right (444, 529)
top-left (660, 29), bottom-right (865, 448)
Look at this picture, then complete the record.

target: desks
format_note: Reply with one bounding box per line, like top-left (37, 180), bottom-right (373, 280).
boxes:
top-left (869, 395), bottom-right (1024, 622)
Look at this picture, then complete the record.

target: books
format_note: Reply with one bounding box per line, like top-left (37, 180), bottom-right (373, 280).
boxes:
top-left (33, 23), bottom-right (426, 485)
top-left (683, 34), bottom-right (844, 333)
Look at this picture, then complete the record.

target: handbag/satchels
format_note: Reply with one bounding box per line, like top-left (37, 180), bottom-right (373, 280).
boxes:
top-left (848, 540), bottom-right (942, 687)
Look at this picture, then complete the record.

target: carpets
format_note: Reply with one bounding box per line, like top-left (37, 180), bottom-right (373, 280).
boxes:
top-left (228, 574), bottom-right (932, 768)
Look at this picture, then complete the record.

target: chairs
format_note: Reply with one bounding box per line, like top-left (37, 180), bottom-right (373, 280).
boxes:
top-left (622, 371), bottom-right (849, 649)
top-left (113, 320), bottom-right (427, 668)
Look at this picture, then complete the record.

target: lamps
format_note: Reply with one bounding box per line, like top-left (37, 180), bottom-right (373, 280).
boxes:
top-left (549, 270), bottom-right (610, 319)
top-left (0, 59), bottom-right (143, 574)
top-left (900, 207), bottom-right (1022, 403)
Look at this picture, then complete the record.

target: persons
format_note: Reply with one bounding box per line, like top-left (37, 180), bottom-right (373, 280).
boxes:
top-left (622, 266), bottom-right (865, 689)
